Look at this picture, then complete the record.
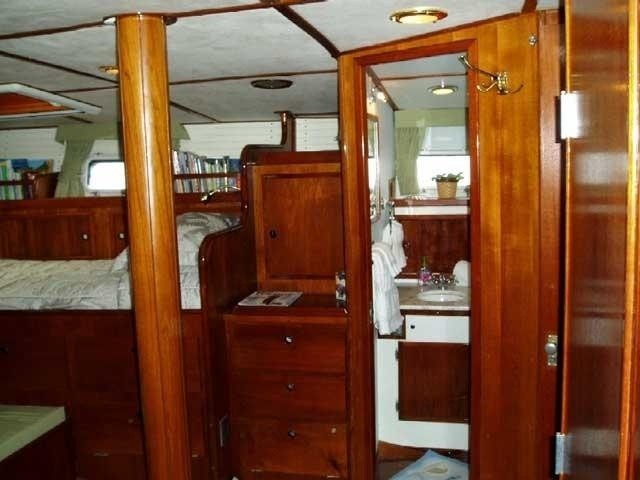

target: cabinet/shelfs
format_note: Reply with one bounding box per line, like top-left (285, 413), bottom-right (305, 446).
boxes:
top-left (1, 191), bottom-right (241, 260)
top-left (240, 151), bottom-right (370, 296)
top-left (217, 296), bottom-right (373, 479)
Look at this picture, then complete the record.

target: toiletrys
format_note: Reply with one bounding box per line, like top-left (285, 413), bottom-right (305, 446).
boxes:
top-left (418, 255), bottom-right (433, 287)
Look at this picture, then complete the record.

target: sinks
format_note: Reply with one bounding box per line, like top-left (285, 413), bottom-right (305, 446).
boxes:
top-left (417, 289), bottom-right (467, 303)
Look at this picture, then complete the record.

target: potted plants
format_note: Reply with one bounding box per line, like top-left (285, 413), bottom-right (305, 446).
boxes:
top-left (431, 171), bottom-right (464, 201)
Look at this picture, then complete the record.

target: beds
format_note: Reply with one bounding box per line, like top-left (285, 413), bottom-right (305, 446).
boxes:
top-left (0, 222), bottom-right (244, 480)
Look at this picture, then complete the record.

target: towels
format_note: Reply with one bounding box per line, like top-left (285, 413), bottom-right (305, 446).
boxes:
top-left (371, 220), bottom-right (408, 337)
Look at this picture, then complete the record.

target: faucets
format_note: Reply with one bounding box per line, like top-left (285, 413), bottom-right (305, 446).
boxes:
top-left (438, 271), bottom-right (454, 290)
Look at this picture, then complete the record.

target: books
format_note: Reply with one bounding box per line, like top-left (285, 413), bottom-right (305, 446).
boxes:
top-left (172, 148), bottom-right (241, 193)
top-left (0, 157), bottom-right (54, 201)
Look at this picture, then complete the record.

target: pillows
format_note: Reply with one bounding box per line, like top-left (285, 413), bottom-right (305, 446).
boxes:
top-left (108, 212), bottom-right (242, 275)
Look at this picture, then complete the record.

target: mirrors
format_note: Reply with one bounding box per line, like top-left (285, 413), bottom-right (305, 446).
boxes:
top-left (366, 114), bottom-right (382, 223)
